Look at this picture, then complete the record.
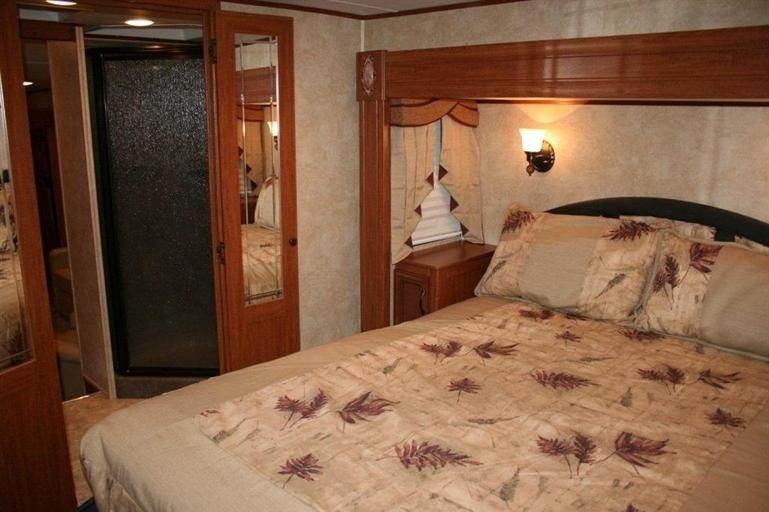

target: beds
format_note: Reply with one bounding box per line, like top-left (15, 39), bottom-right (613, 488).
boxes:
top-left (240, 174), bottom-right (281, 305)
top-left (79, 194), bottom-right (769, 512)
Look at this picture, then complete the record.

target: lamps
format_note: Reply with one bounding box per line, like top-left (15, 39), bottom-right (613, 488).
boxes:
top-left (516, 125), bottom-right (561, 177)
top-left (266, 119), bottom-right (279, 153)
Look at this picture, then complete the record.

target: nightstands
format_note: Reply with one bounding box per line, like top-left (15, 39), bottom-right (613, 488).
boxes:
top-left (390, 235), bottom-right (498, 324)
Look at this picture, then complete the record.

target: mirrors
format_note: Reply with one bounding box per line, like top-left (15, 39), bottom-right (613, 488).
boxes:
top-left (0, 90), bottom-right (37, 376)
top-left (229, 30), bottom-right (287, 307)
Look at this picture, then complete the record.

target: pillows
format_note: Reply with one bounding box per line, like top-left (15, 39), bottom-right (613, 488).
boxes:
top-left (254, 176), bottom-right (282, 233)
top-left (474, 203), bottom-right (663, 331)
top-left (633, 227), bottom-right (769, 363)
